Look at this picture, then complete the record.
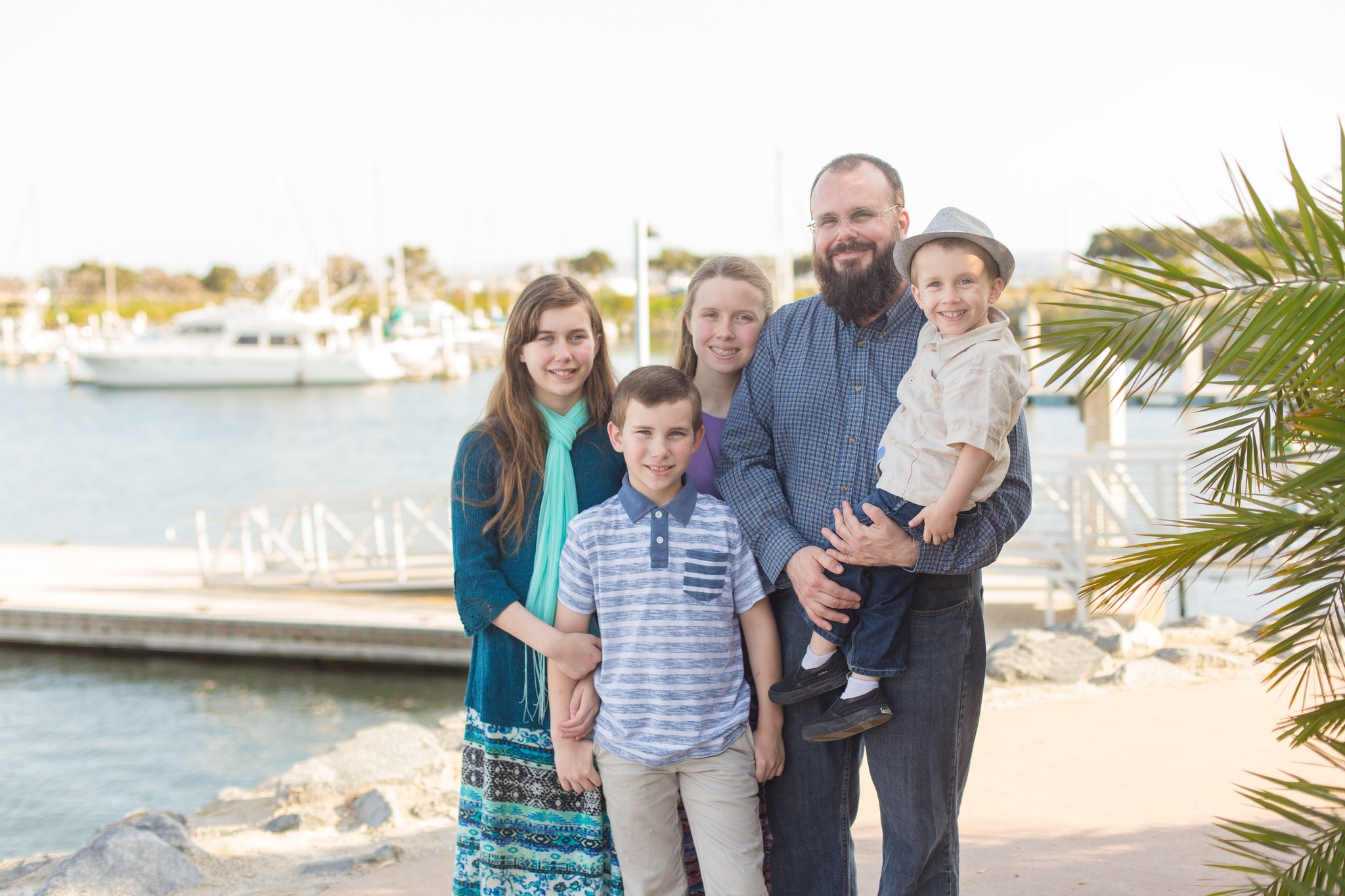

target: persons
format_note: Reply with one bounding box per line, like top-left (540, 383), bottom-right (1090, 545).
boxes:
top-left (676, 256), bottom-right (776, 896)
top-left (450, 275), bottom-right (623, 896)
top-left (768, 206), bottom-right (1032, 742)
top-left (714, 153), bottom-right (1030, 896)
top-left (547, 365), bottom-right (787, 896)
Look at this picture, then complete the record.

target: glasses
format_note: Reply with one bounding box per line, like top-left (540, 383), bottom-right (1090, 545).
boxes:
top-left (807, 205), bottom-right (901, 238)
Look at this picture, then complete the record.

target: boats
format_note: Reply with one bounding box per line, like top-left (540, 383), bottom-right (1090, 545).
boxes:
top-left (67, 257), bottom-right (508, 386)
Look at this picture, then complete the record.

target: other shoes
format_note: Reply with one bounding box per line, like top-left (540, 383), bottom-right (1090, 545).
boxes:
top-left (768, 650), bottom-right (853, 705)
top-left (802, 687), bottom-right (893, 743)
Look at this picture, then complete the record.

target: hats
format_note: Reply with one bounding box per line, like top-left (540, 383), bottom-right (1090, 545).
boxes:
top-left (893, 207), bottom-right (1015, 292)
